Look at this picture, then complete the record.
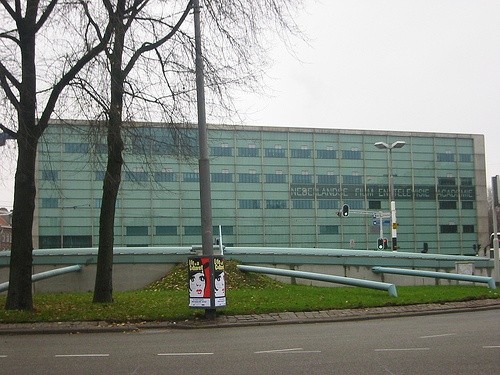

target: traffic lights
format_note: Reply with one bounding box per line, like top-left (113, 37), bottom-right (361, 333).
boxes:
top-left (377, 238), bottom-right (383, 250)
top-left (383, 238), bottom-right (387, 249)
top-left (342, 204), bottom-right (348, 216)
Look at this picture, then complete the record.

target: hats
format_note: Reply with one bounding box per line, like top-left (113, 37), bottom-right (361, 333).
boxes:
top-left (212, 257), bottom-right (224, 278)
top-left (188, 257), bottom-right (209, 277)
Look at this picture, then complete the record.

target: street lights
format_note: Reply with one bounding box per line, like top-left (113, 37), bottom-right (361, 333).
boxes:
top-left (374, 140), bottom-right (406, 251)
